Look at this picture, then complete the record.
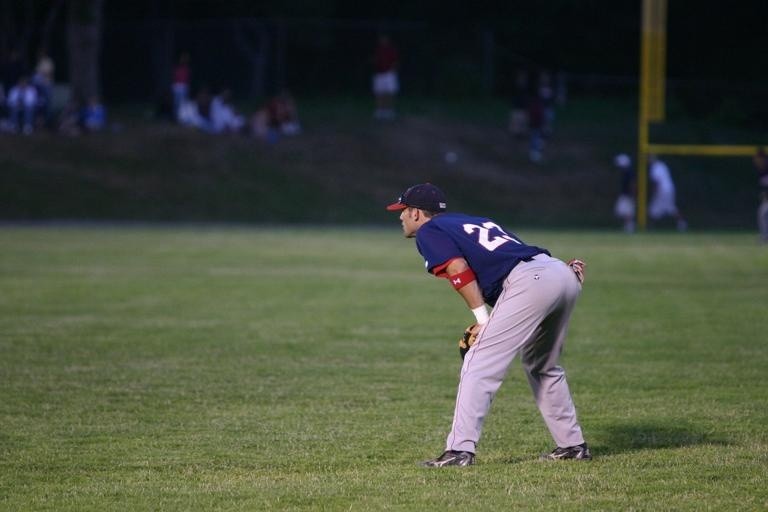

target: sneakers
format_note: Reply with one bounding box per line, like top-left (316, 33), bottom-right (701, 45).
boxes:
top-left (421, 452), bottom-right (475, 466)
top-left (540, 442), bottom-right (593, 460)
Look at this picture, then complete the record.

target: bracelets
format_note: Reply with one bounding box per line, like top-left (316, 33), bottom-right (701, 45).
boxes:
top-left (472, 301), bottom-right (490, 323)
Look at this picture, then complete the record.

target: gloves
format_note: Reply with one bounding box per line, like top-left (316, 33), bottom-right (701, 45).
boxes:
top-left (568, 257), bottom-right (586, 284)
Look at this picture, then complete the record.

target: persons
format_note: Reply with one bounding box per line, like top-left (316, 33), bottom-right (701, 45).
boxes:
top-left (3, 50), bottom-right (312, 133)
top-left (614, 151), bottom-right (638, 230)
top-left (386, 182), bottom-right (593, 465)
top-left (647, 154), bottom-right (689, 230)
top-left (751, 146), bottom-right (768, 234)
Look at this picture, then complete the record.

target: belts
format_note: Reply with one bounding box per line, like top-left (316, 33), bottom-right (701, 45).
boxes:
top-left (522, 257), bottom-right (535, 262)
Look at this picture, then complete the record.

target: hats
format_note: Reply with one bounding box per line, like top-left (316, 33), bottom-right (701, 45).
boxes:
top-left (386, 184), bottom-right (446, 212)
